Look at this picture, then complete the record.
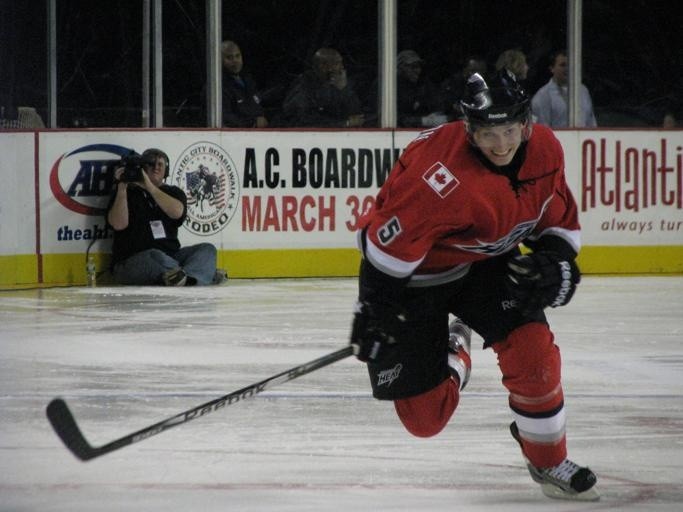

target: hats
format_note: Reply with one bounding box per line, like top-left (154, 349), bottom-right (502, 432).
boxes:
top-left (396, 49), bottom-right (422, 65)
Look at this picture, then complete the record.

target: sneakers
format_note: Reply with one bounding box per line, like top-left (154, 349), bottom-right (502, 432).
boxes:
top-left (214, 269), bottom-right (228, 284)
top-left (164, 268), bottom-right (189, 285)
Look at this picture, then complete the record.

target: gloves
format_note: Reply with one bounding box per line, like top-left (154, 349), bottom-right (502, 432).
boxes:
top-left (422, 111), bottom-right (448, 128)
top-left (350, 302), bottom-right (401, 364)
top-left (509, 249), bottom-right (581, 309)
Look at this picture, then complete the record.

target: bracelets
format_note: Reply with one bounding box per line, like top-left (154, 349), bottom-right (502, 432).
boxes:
top-left (345, 116), bottom-right (351, 128)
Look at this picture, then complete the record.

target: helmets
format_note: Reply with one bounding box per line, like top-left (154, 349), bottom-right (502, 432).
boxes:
top-left (461, 67), bottom-right (532, 146)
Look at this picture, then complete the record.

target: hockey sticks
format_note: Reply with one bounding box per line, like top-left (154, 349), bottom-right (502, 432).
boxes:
top-left (45, 346), bottom-right (353, 463)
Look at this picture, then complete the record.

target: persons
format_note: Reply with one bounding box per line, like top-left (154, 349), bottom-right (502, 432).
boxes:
top-left (106, 146), bottom-right (227, 288)
top-left (199, 40), bottom-right (267, 129)
top-left (361, 49), bottom-right (448, 129)
top-left (494, 50), bottom-right (530, 84)
top-left (530, 48), bottom-right (598, 130)
top-left (282, 47), bottom-right (366, 129)
top-left (353, 58), bottom-right (597, 495)
top-left (442, 56), bottom-right (490, 118)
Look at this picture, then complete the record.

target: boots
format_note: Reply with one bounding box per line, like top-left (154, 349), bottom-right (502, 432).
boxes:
top-left (448, 318), bottom-right (472, 391)
top-left (509, 421), bottom-right (597, 493)
